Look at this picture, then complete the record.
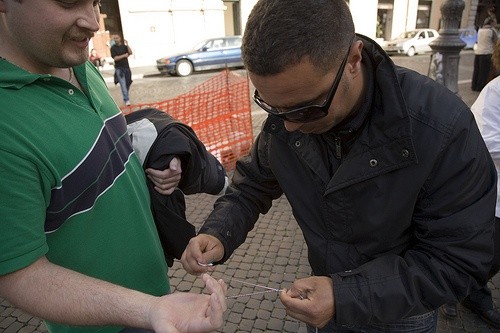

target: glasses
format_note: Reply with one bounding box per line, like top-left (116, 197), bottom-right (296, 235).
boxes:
top-left (252, 34), bottom-right (356, 123)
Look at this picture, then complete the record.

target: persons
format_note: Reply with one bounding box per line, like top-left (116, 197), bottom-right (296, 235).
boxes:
top-left (439, 18), bottom-right (500, 330)
top-left (89, 33), bottom-right (132, 106)
top-left (181, 1), bottom-right (500, 333)
top-left (0, 0), bottom-right (226, 333)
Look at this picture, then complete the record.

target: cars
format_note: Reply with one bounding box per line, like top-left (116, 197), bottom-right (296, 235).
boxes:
top-left (459, 28), bottom-right (478, 51)
top-left (382, 28), bottom-right (440, 56)
top-left (156, 35), bottom-right (246, 77)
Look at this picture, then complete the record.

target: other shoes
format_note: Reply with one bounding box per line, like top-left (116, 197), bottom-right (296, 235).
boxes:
top-left (125, 101), bottom-right (131, 106)
